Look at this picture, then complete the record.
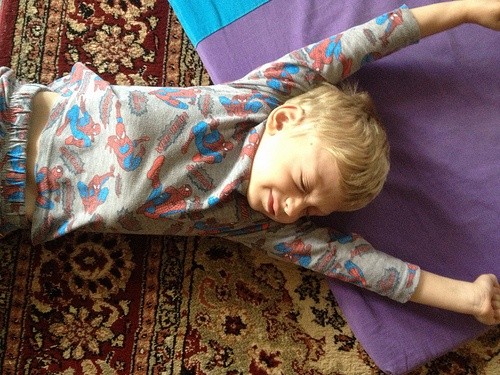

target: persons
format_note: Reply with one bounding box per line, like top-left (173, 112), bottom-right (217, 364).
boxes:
top-left (0, 0), bottom-right (500, 325)
